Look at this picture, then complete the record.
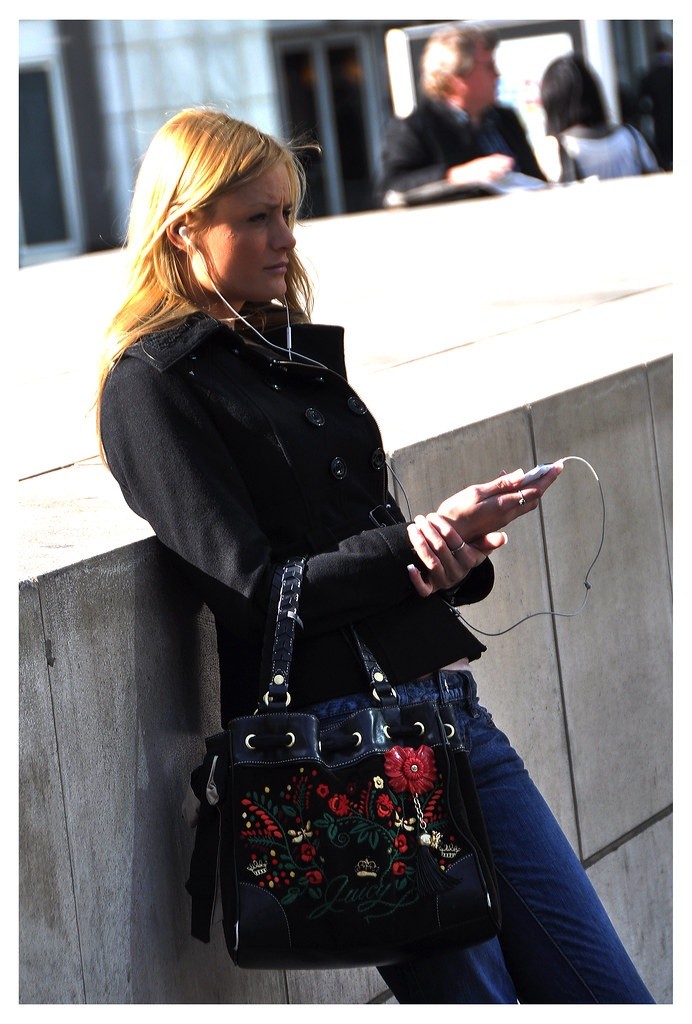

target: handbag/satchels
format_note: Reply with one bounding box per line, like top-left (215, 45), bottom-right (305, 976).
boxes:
top-left (189, 558), bottom-right (503, 971)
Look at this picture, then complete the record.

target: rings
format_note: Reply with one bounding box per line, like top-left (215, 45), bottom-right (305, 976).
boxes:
top-left (518, 489), bottom-right (526, 506)
top-left (451, 541), bottom-right (465, 555)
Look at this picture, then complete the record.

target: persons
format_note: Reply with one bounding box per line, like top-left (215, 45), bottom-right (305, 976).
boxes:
top-left (92, 105), bottom-right (657, 1004)
top-left (374, 23), bottom-right (665, 209)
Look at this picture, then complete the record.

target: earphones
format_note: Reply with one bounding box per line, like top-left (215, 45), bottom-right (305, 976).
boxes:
top-left (179, 225), bottom-right (196, 249)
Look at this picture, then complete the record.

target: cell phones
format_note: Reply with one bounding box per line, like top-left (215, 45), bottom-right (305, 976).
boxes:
top-left (514, 463), bottom-right (564, 488)
